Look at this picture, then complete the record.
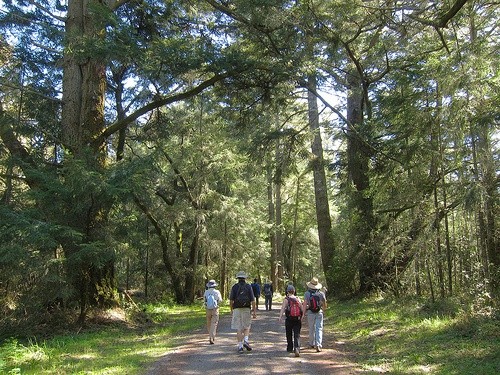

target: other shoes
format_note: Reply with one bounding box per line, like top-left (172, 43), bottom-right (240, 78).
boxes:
top-left (210, 337), bottom-right (214, 344)
top-left (267, 309), bottom-right (272, 311)
top-left (306, 342), bottom-right (314, 348)
top-left (238, 347), bottom-right (244, 353)
top-left (316, 346), bottom-right (322, 352)
top-left (243, 339), bottom-right (253, 351)
top-left (294, 347), bottom-right (300, 357)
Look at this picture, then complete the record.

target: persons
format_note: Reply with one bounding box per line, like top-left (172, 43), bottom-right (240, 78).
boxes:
top-left (230, 271), bottom-right (257, 352)
top-left (303, 277), bottom-right (327, 351)
top-left (265, 280), bottom-right (274, 311)
top-left (279, 281), bottom-right (303, 357)
top-left (251, 278), bottom-right (261, 311)
top-left (203, 279), bottom-right (223, 344)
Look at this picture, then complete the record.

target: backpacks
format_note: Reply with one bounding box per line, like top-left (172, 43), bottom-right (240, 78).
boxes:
top-left (252, 284), bottom-right (258, 297)
top-left (206, 290), bottom-right (219, 310)
top-left (264, 284), bottom-right (271, 297)
top-left (285, 296), bottom-right (303, 320)
top-left (308, 290), bottom-right (321, 314)
top-left (235, 283), bottom-right (251, 308)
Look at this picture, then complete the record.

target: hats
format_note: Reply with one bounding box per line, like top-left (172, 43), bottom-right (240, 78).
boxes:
top-left (266, 280), bottom-right (272, 284)
top-left (306, 277), bottom-right (323, 290)
top-left (206, 280), bottom-right (219, 287)
top-left (235, 271), bottom-right (248, 279)
